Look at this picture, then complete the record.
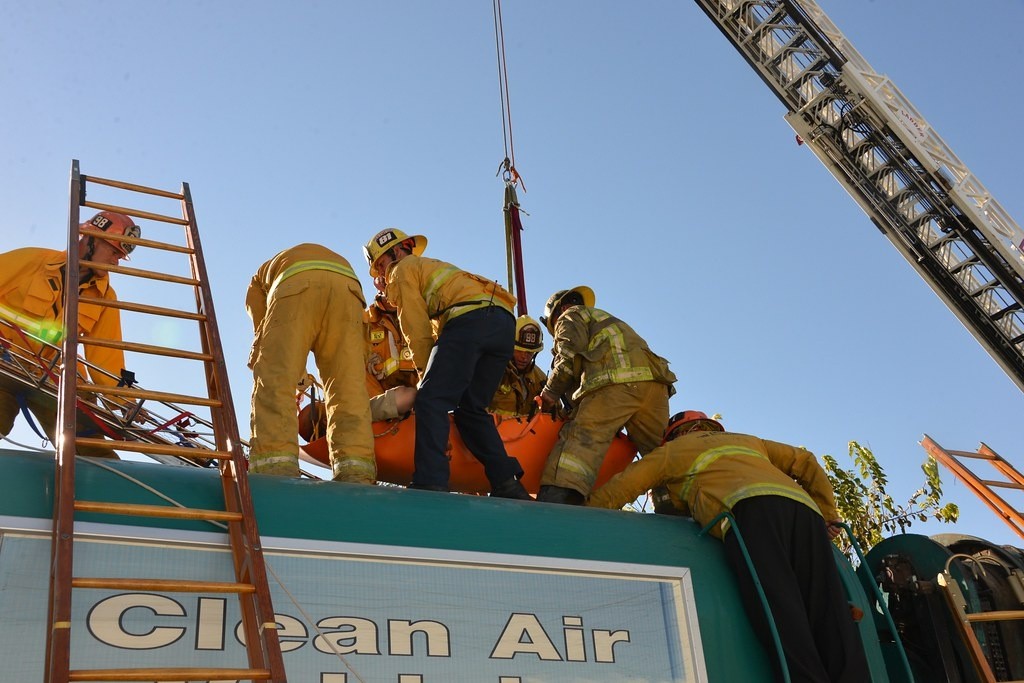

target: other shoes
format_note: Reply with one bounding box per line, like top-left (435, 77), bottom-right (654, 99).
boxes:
top-left (406, 455), bottom-right (452, 492)
top-left (484, 458), bottom-right (532, 500)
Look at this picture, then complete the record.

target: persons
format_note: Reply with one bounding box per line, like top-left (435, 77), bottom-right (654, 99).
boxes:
top-left (485, 315), bottom-right (563, 415)
top-left (585, 409), bottom-right (870, 683)
top-left (298, 385), bottom-right (416, 443)
top-left (245, 244), bottom-right (378, 484)
top-left (363, 228), bottom-right (534, 500)
top-left (0, 212), bottom-right (150, 460)
top-left (535, 286), bottom-right (691, 517)
top-left (364, 287), bottom-right (440, 392)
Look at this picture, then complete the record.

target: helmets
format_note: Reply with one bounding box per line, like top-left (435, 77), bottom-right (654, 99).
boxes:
top-left (77, 209), bottom-right (141, 260)
top-left (367, 228), bottom-right (428, 277)
top-left (540, 285), bottom-right (596, 338)
top-left (513, 314), bottom-right (544, 353)
top-left (662, 410), bottom-right (726, 444)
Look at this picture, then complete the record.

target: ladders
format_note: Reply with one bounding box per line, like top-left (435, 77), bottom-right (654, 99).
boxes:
top-left (916, 433), bottom-right (1024, 541)
top-left (44, 158), bottom-right (290, 683)
top-left (691, 0), bottom-right (1024, 394)
